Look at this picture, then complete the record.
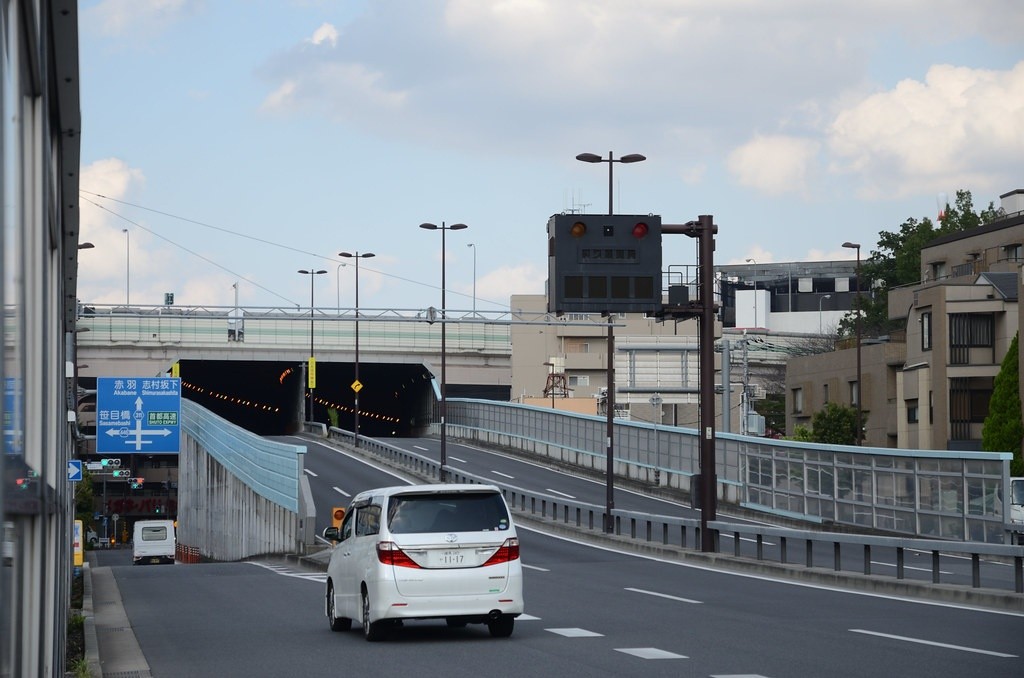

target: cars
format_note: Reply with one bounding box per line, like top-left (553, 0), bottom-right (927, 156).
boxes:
top-left (85, 525), bottom-right (98, 544)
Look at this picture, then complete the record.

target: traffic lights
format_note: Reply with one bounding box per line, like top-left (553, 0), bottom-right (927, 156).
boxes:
top-left (575, 149), bottom-right (649, 535)
top-left (101, 459), bottom-right (121, 467)
top-left (113, 470), bottom-right (131, 477)
top-left (419, 221), bottom-right (468, 483)
top-left (127, 478), bottom-right (144, 489)
top-left (545, 212), bottom-right (664, 246)
top-left (154, 504), bottom-right (160, 513)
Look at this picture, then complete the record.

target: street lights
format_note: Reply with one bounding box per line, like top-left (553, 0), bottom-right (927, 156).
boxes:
top-left (466, 243), bottom-right (476, 319)
top-left (818, 294), bottom-right (832, 334)
top-left (541, 361), bottom-right (556, 410)
top-left (298, 268), bottom-right (327, 434)
top-left (338, 251), bottom-right (376, 448)
top-left (122, 227), bottom-right (130, 309)
top-left (843, 240), bottom-right (864, 506)
top-left (746, 258), bottom-right (758, 329)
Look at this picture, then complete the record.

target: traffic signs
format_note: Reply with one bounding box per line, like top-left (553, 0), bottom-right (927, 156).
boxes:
top-left (96, 377), bottom-right (182, 454)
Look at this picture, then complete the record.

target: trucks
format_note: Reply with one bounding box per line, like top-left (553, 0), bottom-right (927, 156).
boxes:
top-left (132, 519), bottom-right (177, 565)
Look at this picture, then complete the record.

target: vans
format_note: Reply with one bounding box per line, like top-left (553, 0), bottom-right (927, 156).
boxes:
top-left (322, 482), bottom-right (525, 641)
top-left (992, 476), bottom-right (1024, 544)
